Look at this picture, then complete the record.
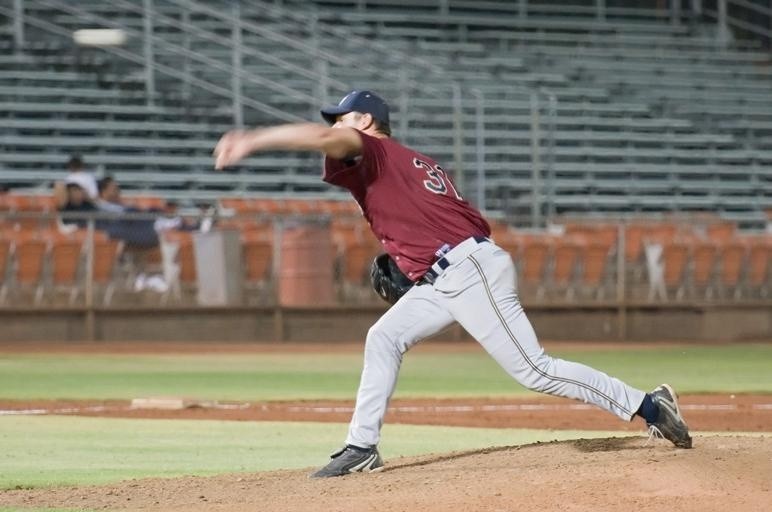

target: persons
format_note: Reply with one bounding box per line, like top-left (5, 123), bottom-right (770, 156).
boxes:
top-left (57, 153), bottom-right (216, 290)
top-left (213, 87), bottom-right (693, 480)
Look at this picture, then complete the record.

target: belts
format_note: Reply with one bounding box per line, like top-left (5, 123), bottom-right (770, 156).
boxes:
top-left (421, 236), bottom-right (487, 284)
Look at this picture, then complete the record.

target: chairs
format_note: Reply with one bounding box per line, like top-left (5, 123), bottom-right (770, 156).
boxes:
top-left (4, 198), bottom-right (762, 319)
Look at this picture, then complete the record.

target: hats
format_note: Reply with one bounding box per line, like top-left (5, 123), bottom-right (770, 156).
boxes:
top-left (320, 90), bottom-right (389, 125)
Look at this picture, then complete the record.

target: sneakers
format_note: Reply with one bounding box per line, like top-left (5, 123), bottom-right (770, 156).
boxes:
top-left (647, 384), bottom-right (692, 449)
top-left (310, 446), bottom-right (382, 478)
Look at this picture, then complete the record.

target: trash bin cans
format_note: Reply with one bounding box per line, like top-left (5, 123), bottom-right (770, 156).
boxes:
top-left (190, 226), bottom-right (248, 309)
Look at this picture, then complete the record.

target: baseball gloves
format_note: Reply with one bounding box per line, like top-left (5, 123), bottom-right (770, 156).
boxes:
top-left (370, 254), bottom-right (413, 303)
